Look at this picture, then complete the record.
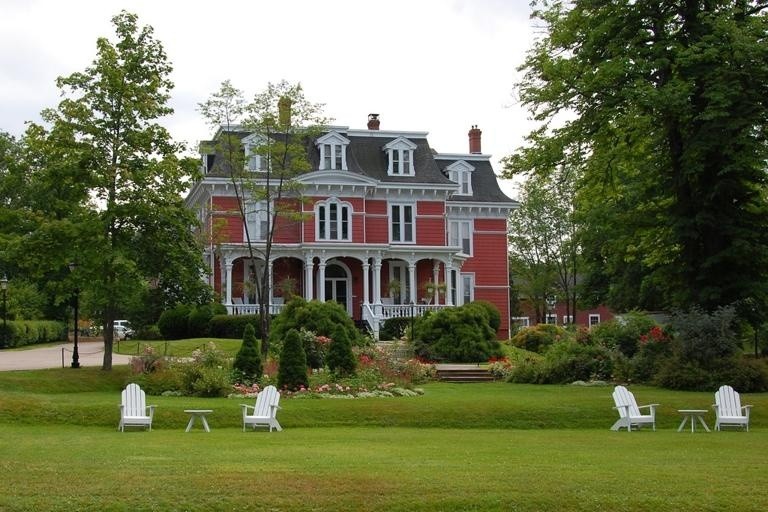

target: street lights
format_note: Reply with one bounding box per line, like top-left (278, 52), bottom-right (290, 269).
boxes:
top-left (68, 250), bottom-right (82, 368)
top-left (409, 299), bottom-right (415, 340)
top-left (0, 273), bottom-right (10, 350)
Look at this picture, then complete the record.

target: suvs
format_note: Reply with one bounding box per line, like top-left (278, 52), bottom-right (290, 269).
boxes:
top-left (112, 319), bottom-right (133, 330)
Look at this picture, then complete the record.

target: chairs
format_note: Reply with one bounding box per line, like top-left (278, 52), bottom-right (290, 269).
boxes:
top-left (381, 296), bottom-right (433, 305)
top-left (233, 296), bottom-right (285, 305)
top-left (116, 382), bottom-right (155, 433)
top-left (239, 385), bottom-right (285, 433)
top-left (711, 385), bottom-right (754, 433)
top-left (609, 384), bottom-right (660, 432)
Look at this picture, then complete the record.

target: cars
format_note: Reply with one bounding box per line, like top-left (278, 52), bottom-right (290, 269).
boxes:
top-left (88, 325), bottom-right (135, 341)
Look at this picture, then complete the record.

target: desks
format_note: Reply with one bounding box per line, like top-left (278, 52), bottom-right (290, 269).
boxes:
top-left (676, 409), bottom-right (711, 433)
top-left (183, 409), bottom-right (214, 433)
